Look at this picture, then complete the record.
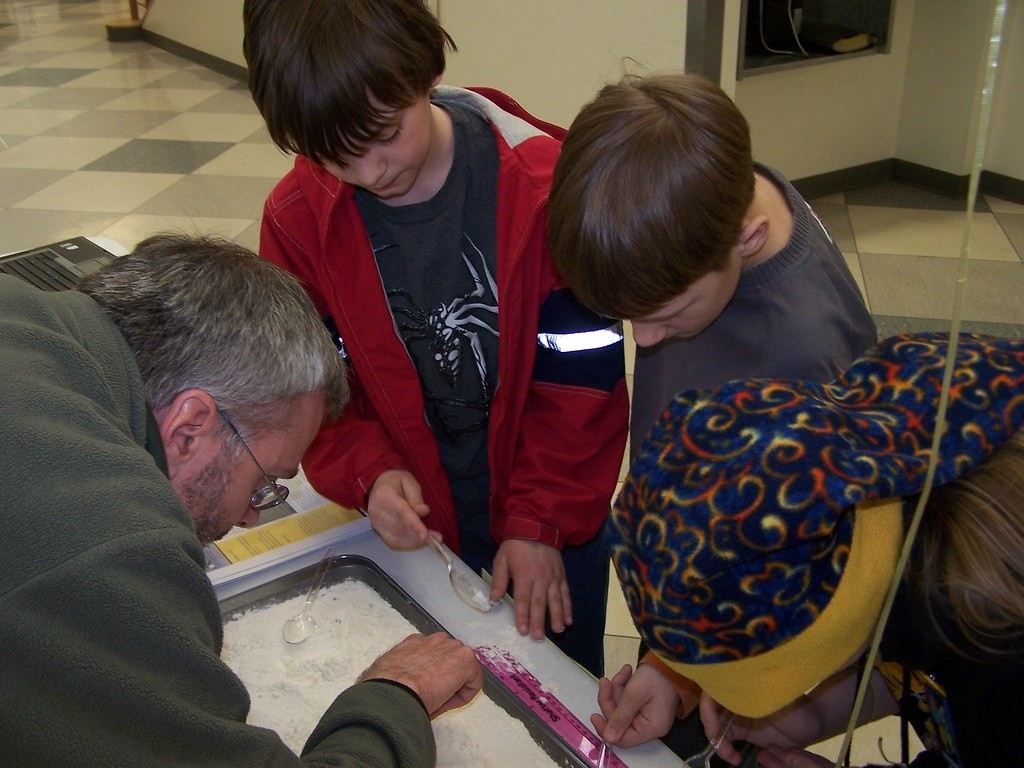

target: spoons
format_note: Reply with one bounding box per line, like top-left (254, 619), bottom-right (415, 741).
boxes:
top-left (283, 547), bottom-right (337, 644)
top-left (681, 711), bottom-right (736, 768)
top-left (426, 533), bottom-right (491, 612)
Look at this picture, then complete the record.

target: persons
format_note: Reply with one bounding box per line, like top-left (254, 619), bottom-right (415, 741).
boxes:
top-left (0, 228), bottom-right (483, 768)
top-left (242, 0), bottom-right (630, 677)
top-left (550, 74), bottom-right (877, 749)
top-left (604, 332), bottom-right (1024, 768)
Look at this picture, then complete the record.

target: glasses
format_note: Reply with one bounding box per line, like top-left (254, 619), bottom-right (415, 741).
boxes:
top-left (212, 403), bottom-right (289, 512)
